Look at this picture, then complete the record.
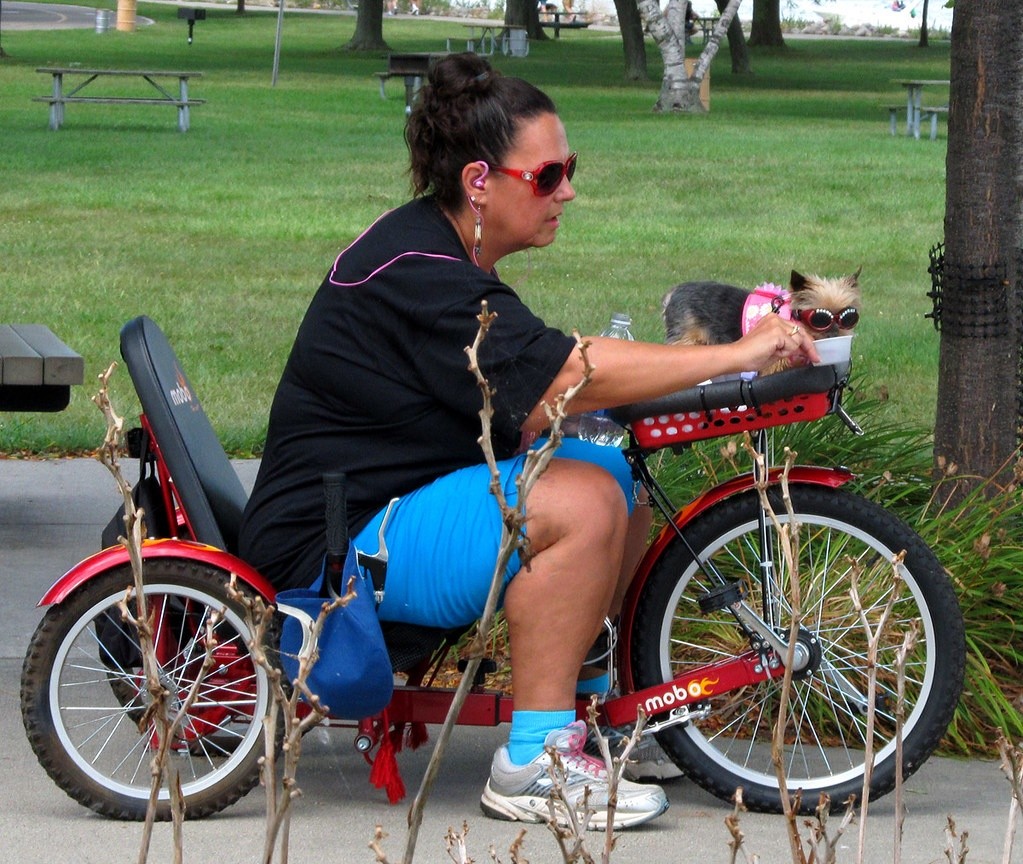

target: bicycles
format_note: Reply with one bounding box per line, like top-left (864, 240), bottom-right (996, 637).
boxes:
top-left (19, 312), bottom-right (967, 821)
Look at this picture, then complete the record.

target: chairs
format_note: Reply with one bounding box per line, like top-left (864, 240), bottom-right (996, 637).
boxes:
top-left (118, 309), bottom-right (454, 659)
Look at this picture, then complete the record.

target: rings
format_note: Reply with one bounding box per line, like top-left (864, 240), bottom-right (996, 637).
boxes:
top-left (789, 326), bottom-right (800, 335)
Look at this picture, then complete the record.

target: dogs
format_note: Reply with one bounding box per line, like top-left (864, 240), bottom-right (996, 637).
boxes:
top-left (659, 264), bottom-right (863, 379)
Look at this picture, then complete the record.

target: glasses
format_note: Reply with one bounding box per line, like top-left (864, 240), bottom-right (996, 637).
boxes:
top-left (794, 306), bottom-right (859, 332)
top-left (479, 151), bottom-right (577, 197)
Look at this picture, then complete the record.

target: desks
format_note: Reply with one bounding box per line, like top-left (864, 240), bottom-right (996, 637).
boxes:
top-left (687, 14), bottom-right (721, 45)
top-left (885, 76), bottom-right (950, 142)
top-left (534, 10), bottom-right (591, 41)
top-left (30, 64), bottom-right (210, 133)
top-left (458, 20), bottom-right (529, 56)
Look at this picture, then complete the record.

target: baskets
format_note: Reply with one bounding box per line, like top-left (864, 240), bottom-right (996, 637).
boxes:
top-left (609, 360), bottom-right (852, 448)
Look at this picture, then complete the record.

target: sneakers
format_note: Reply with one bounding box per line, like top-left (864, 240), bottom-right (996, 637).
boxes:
top-left (583, 723), bottom-right (688, 783)
top-left (480, 720), bottom-right (671, 831)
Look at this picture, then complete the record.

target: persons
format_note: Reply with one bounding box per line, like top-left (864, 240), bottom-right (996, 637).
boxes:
top-left (239, 50), bottom-right (820, 830)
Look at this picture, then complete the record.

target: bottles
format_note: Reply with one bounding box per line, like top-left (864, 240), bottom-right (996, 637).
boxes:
top-left (580, 308), bottom-right (638, 449)
